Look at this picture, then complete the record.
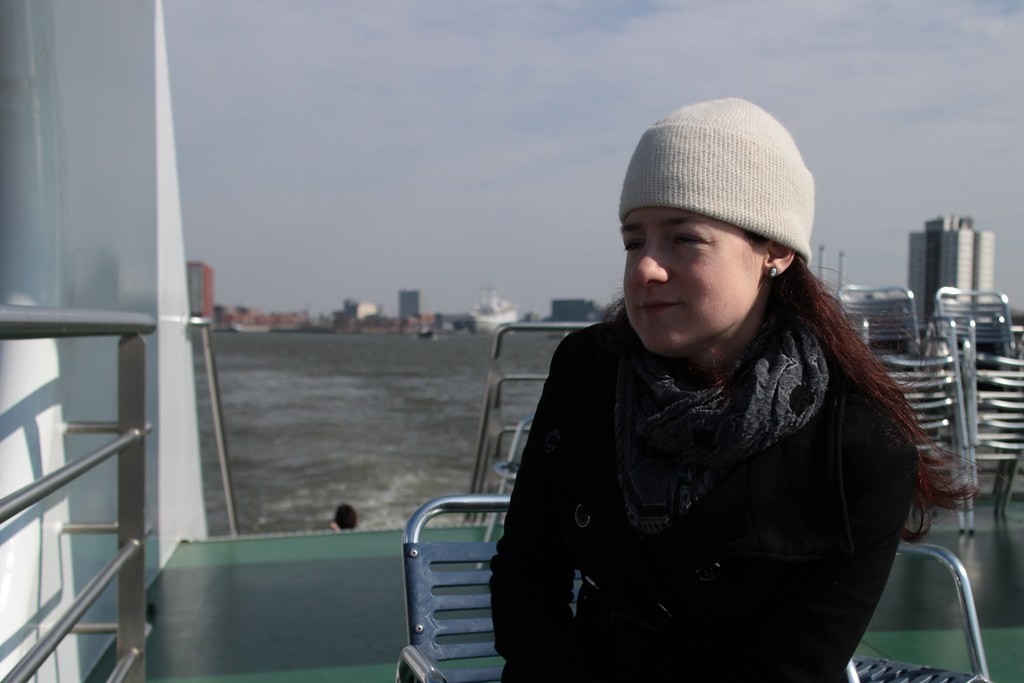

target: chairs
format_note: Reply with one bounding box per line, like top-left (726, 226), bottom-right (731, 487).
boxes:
top-left (394, 278), bottom-right (1024, 683)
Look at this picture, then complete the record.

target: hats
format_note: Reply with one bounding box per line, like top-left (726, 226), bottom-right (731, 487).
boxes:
top-left (618, 98), bottom-right (814, 269)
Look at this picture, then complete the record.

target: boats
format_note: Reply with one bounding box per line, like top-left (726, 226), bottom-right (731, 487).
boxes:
top-left (466, 286), bottom-right (520, 340)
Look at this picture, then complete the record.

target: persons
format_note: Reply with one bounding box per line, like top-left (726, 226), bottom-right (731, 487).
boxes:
top-left (487, 94), bottom-right (977, 682)
